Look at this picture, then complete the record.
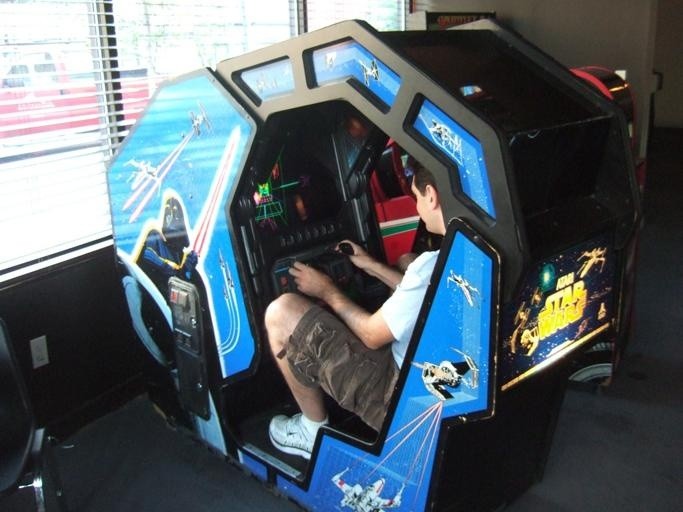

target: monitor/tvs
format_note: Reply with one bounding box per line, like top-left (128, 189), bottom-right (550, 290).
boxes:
top-left (251, 130), bottom-right (351, 256)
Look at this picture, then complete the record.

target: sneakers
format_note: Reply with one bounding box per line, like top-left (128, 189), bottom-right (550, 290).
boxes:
top-left (268, 413), bottom-right (314, 461)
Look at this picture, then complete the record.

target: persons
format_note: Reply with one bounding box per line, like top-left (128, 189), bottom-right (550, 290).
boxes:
top-left (262, 153), bottom-right (444, 462)
